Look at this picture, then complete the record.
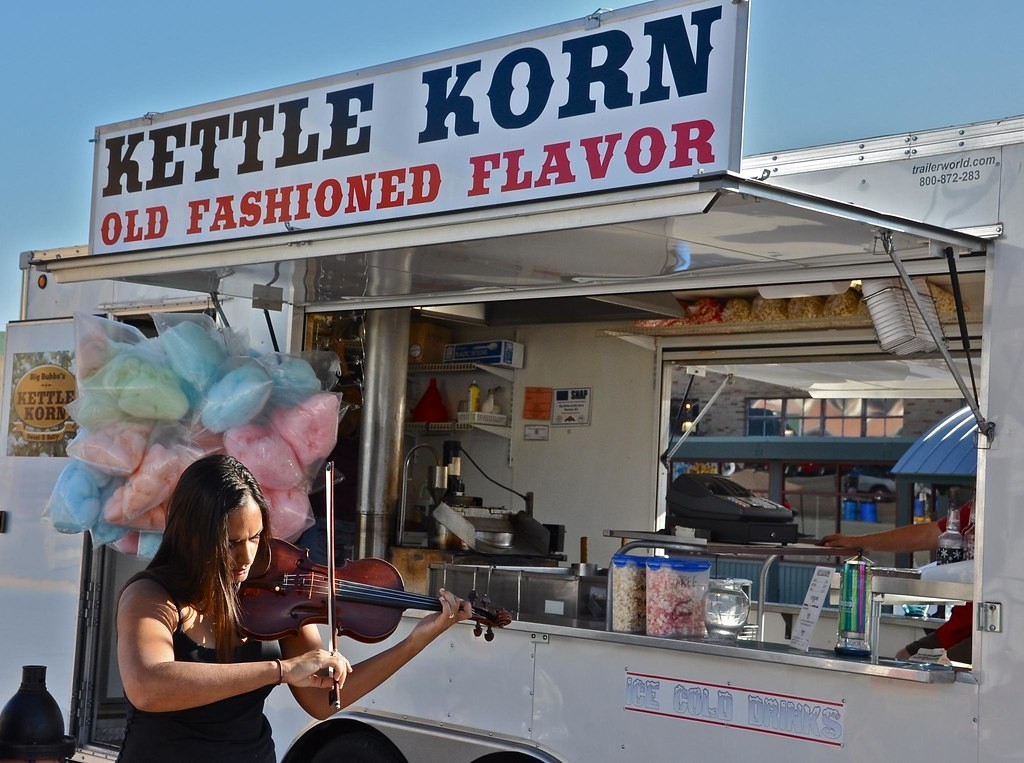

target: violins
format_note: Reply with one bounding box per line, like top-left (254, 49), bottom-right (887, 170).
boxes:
top-left (232, 536), bottom-right (512, 644)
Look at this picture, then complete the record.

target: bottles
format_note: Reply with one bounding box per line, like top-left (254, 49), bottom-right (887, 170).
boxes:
top-left (913, 492), bottom-right (932, 567)
top-left (468, 379), bottom-right (480, 422)
top-left (0, 663), bottom-right (64, 744)
top-left (961, 500), bottom-right (975, 561)
top-left (936, 508), bottom-right (964, 565)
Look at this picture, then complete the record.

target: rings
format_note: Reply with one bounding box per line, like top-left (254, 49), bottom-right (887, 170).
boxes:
top-left (449, 613), bottom-right (454, 618)
top-left (331, 651), bottom-right (336, 655)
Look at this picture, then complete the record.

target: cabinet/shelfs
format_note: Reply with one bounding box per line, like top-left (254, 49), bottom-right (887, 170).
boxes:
top-left (404, 362), bottom-right (517, 467)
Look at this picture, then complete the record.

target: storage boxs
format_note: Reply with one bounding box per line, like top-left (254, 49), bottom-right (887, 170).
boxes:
top-left (445, 340), bottom-right (524, 369)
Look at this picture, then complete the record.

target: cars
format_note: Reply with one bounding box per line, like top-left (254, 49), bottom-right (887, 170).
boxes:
top-left (758, 431), bottom-right (851, 476)
top-left (839, 466), bottom-right (931, 503)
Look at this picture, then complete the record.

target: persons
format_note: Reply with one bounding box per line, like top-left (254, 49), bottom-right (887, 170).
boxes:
top-left (816, 497), bottom-right (973, 664)
top-left (113, 455), bottom-right (473, 763)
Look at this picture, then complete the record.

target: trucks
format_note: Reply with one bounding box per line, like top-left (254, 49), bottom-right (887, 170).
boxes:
top-left (748, 408), bottom-right (798, 436)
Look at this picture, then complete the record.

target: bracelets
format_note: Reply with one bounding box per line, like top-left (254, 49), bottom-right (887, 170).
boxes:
top-left (275, 658), bottom-right (283, 687)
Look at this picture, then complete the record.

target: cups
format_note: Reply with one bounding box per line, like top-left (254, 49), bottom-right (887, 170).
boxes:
top-left (645, 557), bottom-right (713, 642)
top-left (611, 554), bottom-right (648, 635)
top-left (701, 580), bottom-right (751, 647)
